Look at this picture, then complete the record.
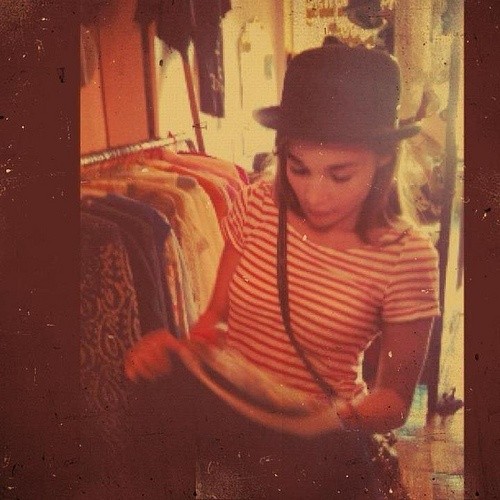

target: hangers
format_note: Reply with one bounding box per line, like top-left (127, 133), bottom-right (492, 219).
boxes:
top-left (80, 133), bottom-right (166, 188)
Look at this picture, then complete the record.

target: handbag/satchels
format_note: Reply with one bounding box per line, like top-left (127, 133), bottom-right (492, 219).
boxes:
top-left (334, 431), bottom-right (406, 500)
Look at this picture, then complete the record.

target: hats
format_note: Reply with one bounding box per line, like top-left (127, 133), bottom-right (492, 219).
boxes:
top-left (251, 44), bottom-right (421, 145)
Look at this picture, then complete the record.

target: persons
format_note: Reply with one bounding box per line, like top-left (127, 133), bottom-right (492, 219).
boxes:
top-left (125, 35), bottom-right (439, 498)
